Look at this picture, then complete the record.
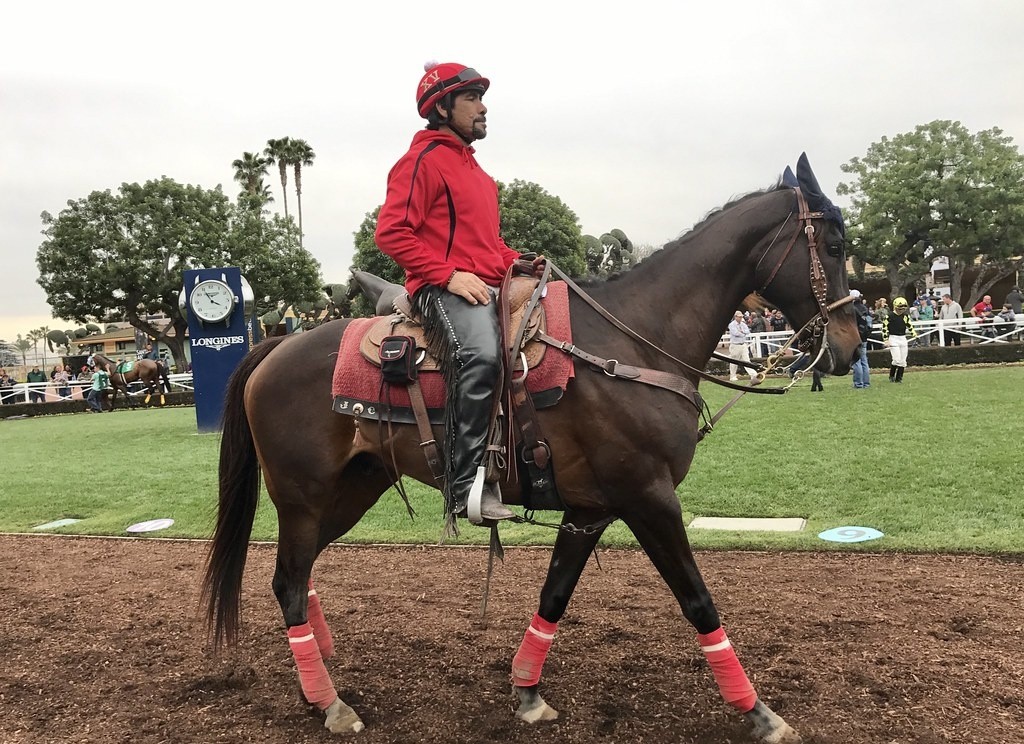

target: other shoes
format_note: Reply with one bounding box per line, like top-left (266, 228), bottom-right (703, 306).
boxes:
top-left (730, 377), bottom-right (737, 381)
top-left (470, 491), bottom-right (515, 520)
top-left (86, 409), bottom-right (95, 413)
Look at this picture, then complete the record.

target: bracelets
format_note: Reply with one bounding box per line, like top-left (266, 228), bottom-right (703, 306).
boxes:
top-left (447, 270), bottom-right (457, 284)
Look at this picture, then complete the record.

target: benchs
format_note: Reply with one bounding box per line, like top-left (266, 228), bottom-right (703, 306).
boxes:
top-left (965, 314), bottom-right (1024, 344)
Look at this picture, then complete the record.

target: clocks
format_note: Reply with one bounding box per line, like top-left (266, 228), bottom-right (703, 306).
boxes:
top-left (190, 280), bottom-right (237, 324)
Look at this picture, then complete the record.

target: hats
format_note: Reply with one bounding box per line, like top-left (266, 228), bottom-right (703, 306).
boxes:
top-left (735, 311), bottom-right (744, 317)
top-left (981, 308), bottom-right (991, 312)
top-left (32, 363), bottom-right (39, 366)
top-left (918, 296), bottom-right (926, 300)
top-left (850, 290), bottom-right (863, 298)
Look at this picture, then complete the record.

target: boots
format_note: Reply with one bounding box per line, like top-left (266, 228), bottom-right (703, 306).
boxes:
top-left (889, 365), bottom-right (896, 381)
top-left (896, 367), bottom-right (904, 382)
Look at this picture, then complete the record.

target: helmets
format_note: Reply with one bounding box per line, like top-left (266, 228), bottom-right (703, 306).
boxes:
top-left (417, 61), bottom-right (491, 118)
top-left (893, 297), bottom-right (906, 311)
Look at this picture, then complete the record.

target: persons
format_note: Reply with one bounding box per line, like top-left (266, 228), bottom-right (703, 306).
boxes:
top-left (86, 363), bottom-right (111, 412)
top-left (374, 59), bottom-right (556, 521)
top-left (51, 364), bottom-right (76, 400)
top-left (77, 366), bottom-right (92, 399)
top-left (144, 344), bottom-right (159, 362)
top-left (0, 369), bottom-right (17, 404)
top-left (729, 285), bottom-right (1024, 389)
top-left (27, 363), bottom-right (47, 402)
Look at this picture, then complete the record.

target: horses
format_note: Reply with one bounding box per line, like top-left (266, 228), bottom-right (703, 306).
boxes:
top-left (87, 353), bottom-right (171, 412)
top-left (346, 266), bottom-right (408, 317)
top-left (192, 150), bottom-right (863, 744)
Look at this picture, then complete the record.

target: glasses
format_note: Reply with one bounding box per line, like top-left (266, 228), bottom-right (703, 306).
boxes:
top-left (456, 67), bottom-right (482, 84)
